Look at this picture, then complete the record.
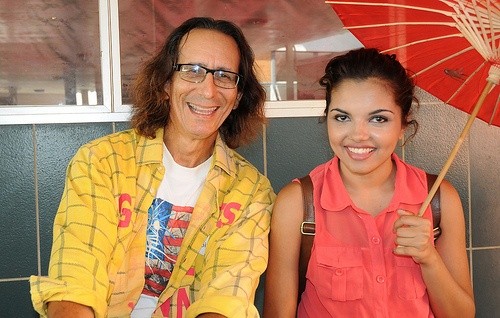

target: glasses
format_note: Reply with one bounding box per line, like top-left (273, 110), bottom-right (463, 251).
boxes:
top-left (172, 64), bottom-right (243, 89)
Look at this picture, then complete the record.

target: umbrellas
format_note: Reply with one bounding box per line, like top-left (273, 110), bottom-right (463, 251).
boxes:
top-left (324, 0), bottom-right (500, 248)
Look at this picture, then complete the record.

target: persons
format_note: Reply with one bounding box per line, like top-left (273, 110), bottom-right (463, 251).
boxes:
top-left (262, 47), bottom-right (476, 317)
top-left (30, 17), bottom-right (277, 317)
